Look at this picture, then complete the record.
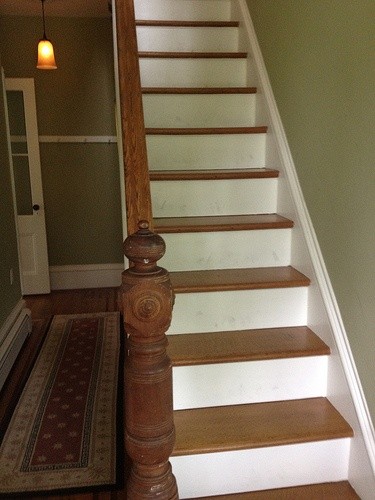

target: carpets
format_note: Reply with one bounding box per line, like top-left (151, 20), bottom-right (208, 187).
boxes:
top-left (1, 309), bottom-right (127, 500)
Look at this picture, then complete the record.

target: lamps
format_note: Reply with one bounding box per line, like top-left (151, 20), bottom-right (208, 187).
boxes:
top-left (34, 0), bottom-right (59, 70)
